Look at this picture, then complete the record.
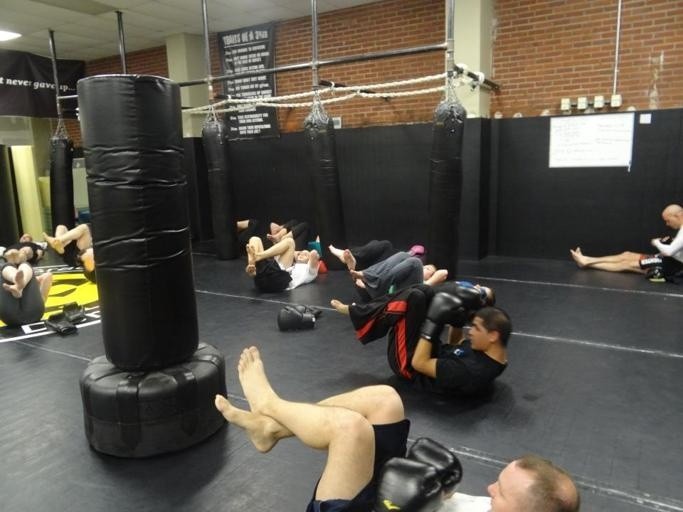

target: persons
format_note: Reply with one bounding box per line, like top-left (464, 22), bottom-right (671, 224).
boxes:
top-left (330, 283), bottom-right (511, 405)
top-left (327, 238), bottom-right (433, 273)
top-left (3, 232), bottom-right (45, 267)
top-left (569, 204), bottom-right (682, 286)
top-left (0, 262), bottom-right (53, 328)
top-left (243, 235), bottom-right (321, 294)
top-left (234, 217), bottom-right (270, 253)
top-left (76, 246), bottom-right (95, 284)
top-left (349, 250), bottom-right (449, 300)
top-left (42, 221), bottom-right (92, 267)
top-left (451, 279), bottom-right (495, 308)
top-left (266, 216), bottom-right (315, 253)
top-left (213, 343), bottom-right (579, 511)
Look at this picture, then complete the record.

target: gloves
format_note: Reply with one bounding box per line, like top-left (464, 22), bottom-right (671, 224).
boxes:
top-left (285, 304), bottom-right (321, 316)
top-left (418, 291), bottom-right (464, 344)
top-left (372, 456), bottom-right (443, 512)
top-left (408, 437), bottom-right (463, 499)
top-left (45, 312), bottom-right (78, 335)
top-left (278, 307), bottom-right (315, 332)
top-left (408, 245), bottom-right (425, 257)
top-left (448, 286), bottom-right (480, 328)
top-left (62, 301), bottom-right (87, 324)
